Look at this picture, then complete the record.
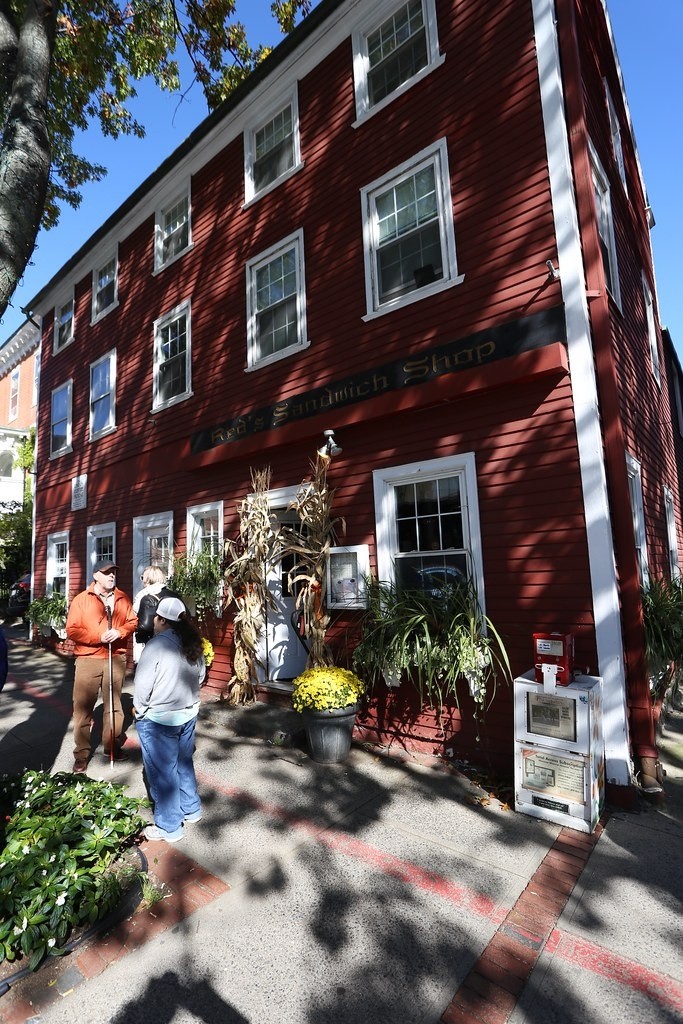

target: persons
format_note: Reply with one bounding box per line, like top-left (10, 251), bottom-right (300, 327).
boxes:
top-left (66, 559), bottom-right (138, 773)
top-left (19, 566), bottom-right (30, 581)
top-left (135, 565), bottom-right (192, 648)
top-left (132, 596), bottom-right (206, 843)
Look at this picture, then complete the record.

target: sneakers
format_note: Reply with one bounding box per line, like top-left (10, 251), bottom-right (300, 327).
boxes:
top-left (105, 750), bottom-right (129, 760)
top-left (186, 814), bottom-right (202, 824)
top-left (144, 825), bottom-right (183, 843)
top-left (73, 759), bottom-right (87, 773)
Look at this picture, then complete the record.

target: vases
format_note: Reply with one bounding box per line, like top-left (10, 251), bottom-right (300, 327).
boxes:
top-left (301, 699), bottom-right (362, 765)
top-left (378, 663), bottom-right (483, 699)
top-left (35, 614), bottom-right (68, 640)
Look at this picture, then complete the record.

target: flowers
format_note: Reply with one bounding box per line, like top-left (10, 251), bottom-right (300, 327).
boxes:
top-left (202, 637), bottom-right (214, 668)
top-left (307, 548), bottom-right (514, 758)
top-left (24, 580), bottom-right (69, 649)
top-left (291, 663), bottom-right (370, 715)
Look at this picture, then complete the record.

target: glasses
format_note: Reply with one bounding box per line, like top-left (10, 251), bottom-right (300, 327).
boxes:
top-left (140, 574), bottom-right (144, 582)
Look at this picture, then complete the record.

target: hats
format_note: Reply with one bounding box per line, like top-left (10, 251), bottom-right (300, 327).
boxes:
top-left (93, 560), bottom-right (120, 573)
top-left (144, 596), bottom-right (187, 622)
